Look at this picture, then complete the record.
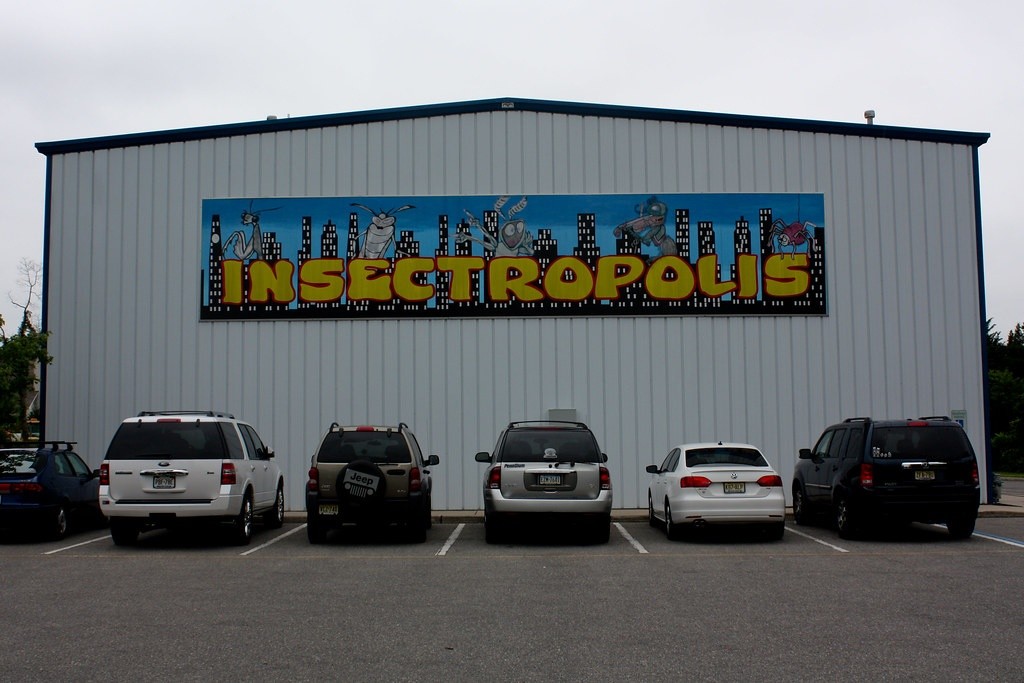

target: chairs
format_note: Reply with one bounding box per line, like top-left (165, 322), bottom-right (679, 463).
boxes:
top-left (560, 442), bottom-right (585, 458)
top-left (896, 439), bottom-right (914, 456)
top-left (501, 441), bottom-right (533, 462)
top-left (385, 444), bottom-right (408, 461)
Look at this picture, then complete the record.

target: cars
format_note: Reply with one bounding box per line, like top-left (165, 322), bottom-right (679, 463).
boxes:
top-left (0, 440), bottom-right (110, 543)
top-left (9, 417), bottom-right (40, 442)
top-left (646, 442), bottom-right (787, 542)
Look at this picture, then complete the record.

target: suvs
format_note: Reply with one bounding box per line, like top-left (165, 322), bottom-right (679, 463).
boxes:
top-left (99, 408), bottom-right (286, 548)
top-left (474, 419), bottom-right (614, 545)
top-left (305, 422), bottom-right (440, 544)
top-left (791, 415), bottom-right (980, 541)
